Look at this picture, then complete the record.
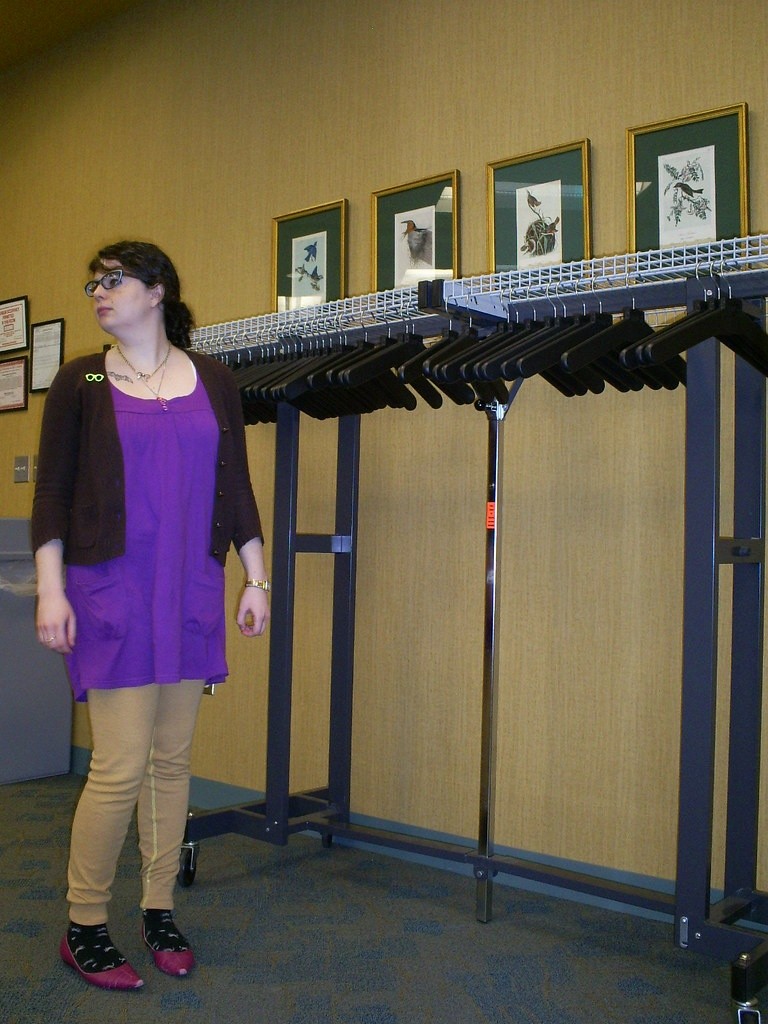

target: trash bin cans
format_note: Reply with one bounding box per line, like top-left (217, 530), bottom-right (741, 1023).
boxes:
top-left (0, 516), bottom-right (73, 787)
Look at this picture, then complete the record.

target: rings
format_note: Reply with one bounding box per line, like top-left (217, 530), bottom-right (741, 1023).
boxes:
top-left (45, 637), bottom-right (55, 643)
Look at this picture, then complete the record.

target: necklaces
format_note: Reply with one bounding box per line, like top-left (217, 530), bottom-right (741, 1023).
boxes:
top-left (116, 340), bottom-right (172, 412)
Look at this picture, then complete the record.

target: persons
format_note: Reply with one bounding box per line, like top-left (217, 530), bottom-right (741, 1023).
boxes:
top-left (32, 240), bottom-right (270, 987)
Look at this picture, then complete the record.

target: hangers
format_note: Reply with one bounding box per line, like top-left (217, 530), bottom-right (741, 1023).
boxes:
top-left (189, 256), bottom-right (767, 427)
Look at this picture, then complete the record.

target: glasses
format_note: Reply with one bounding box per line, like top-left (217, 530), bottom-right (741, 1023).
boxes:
top-left (84, 268), bottom-right (143, 298)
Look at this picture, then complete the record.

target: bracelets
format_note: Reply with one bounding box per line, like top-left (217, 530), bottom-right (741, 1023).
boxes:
top-left (244, 578), bottom-right (271, 593)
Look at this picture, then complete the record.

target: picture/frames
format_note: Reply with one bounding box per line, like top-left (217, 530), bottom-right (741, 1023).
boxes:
top-left (369, 169), bottom-right (461, 293)
top-left (30, 318), bottom-right (64, 393)
top-left (0, 295), bottom-right (30, 355)
top-left (486, 138), bottom-right (592, 274)
top-left (268, 198), bottom-right (349, 314)
top-left (626, 101), bottom-right (751, 284)
top-left (0, 355), bottom-right (29, 412)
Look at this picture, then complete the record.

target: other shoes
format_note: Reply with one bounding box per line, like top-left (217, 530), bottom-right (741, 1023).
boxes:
top-left (141, 921), bottom-right (195, 976)
top-left (60, 936), bottom-right (144, 990)
